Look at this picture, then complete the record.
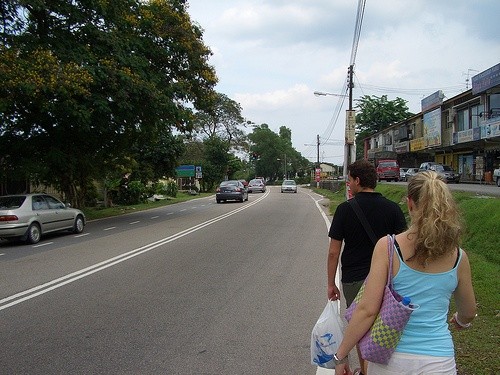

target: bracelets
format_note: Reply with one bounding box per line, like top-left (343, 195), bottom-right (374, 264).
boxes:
top-left (453, 311), bottom-right (478, 328)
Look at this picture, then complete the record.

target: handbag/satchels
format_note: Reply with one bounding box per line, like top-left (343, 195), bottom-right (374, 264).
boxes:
top-left (310, 295), bottom-right (349, 370)
top-left (342, 234), bottom-right (420, 367)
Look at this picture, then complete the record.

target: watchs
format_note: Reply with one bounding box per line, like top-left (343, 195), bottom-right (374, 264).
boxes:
top-left (333, 353), bottom-right (349, 365)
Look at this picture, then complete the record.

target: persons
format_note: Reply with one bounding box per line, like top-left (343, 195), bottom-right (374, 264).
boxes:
top-left (334, 170), bottom-right (477, 375)
top-left (326, 160), bottom-right (409, 375)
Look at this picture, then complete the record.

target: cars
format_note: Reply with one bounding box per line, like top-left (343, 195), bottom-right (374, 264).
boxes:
top-left (375, 160), bottom-right (460, 184)
top-left (239, 176), bottom-right (266, 193)
top-left (0, 193), bottom-right (87, 245)
top-left (281, 179), bottom-right (297, 193)
top-left (215, 180), bottom-right (248, 203)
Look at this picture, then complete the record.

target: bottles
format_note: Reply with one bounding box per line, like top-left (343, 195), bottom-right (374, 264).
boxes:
top-left (399, 297), bottom-right (411, 308)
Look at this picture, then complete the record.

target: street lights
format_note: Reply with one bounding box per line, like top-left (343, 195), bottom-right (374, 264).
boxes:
top-left (303, 143), bottom-right (320, 189)
top-left (277, 158), bottom-right (286, 179)
top-left (313, 91), bottom-right (353, 176)
